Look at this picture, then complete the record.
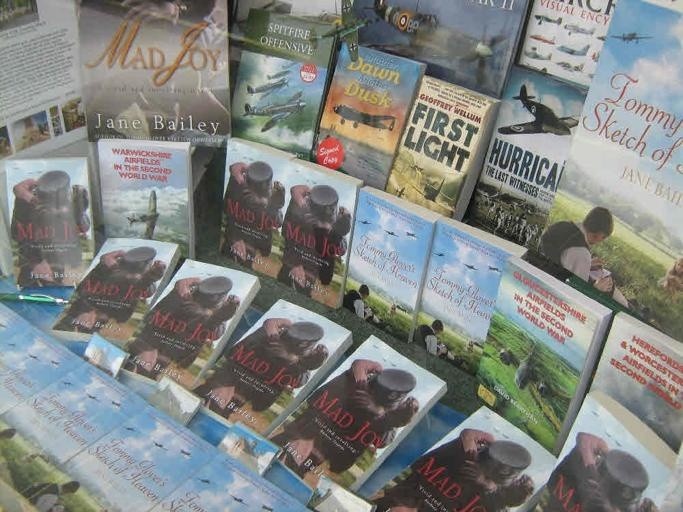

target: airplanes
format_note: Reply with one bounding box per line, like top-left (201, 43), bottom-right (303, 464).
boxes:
top-left (525, 15), bottom-right (606, 78)
top-left (127, 191), bottom-right (160, 239)
top-left (334, 104), bottom-right (396, 132)
top-left (498, 84), bottom-right (579, 135)
top-left (414, 178), bottom-right (454, 209)
top-left (243, 62), bottom-right (307, 131)
top-left (612, 32), bottom-right (652, 44)
top-left (499, 339), bottom-right (547, 396)
top-left (363, 0), bottom-right (504, 76)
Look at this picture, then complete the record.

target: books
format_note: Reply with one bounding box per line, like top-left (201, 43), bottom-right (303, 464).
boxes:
top-left (1, 2), bottom-right (683, 512)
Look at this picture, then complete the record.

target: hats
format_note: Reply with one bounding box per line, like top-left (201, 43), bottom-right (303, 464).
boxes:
top-left (33, 170), bottom-right (73, 209)
top-left (284, 320), bottom-right (325, 352)
top-left (193, 275), bottom-right (234, 308)
top-left (599, 447), bottom-right (649, 504)
top-left (373, 369), bottom-right (416, 408)
top-left (245, 160), bottom-right (273, 191)
top-left (118, 245), bottom-right (158, 274)
top-left (307, 182), bottom-right (338, 226)
top-left (485, 439), bottom-right (533, 478)
